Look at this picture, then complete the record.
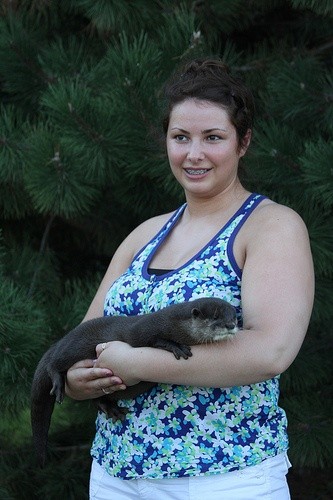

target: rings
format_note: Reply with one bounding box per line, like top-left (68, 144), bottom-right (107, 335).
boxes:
top-left (102, 387), bottom-right (110, 394)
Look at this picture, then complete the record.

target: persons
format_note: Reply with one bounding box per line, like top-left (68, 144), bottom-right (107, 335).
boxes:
top-left (60, 58), bottom-right (316, 500)
